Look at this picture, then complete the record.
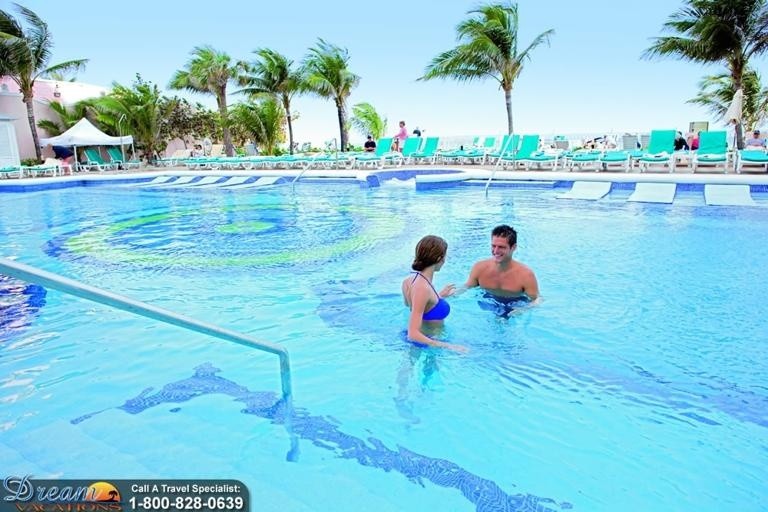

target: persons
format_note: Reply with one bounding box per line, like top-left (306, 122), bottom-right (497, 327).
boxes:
top-left (364, 135), bottom-right (376, 152)
top-left (65, 155), bottom-right (75, 175)
top-left (675, 128), bottom-right (701, 152)
top-left (745, 131), bottom-right (766, 150)
top-left (288, 142), bottom-right (300, 151)
top-left (43, 144), bottom-right (56, 160)
top-left (251, 141), bottom-right (258, 154)
top-left (126, 145), bottom-right (158, 166)
top-left (401, 235), bottom-right (466, 353)
top-left (450, 225), bottom-right (544, 318)
top-left (391, 121), bottom-right (421, 151)
top-left (191, 144), bottom-right (204, 169)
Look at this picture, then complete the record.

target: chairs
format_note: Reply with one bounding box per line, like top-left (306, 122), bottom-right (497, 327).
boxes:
top-left (0, 123), bottom-right (767, 180)
